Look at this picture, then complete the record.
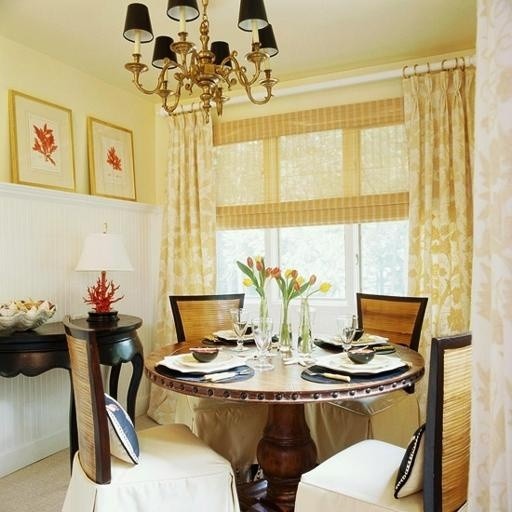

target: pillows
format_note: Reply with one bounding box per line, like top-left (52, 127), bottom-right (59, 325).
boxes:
top-left (394, 421), bottom-right (426, 499)
top-left (104, 393), bottom-right (139, 464)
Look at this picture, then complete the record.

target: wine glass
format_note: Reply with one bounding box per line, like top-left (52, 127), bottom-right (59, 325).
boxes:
top-left (251, 319), bottom-right (273, 371)
top-left (334, 314), bottom-right (358, 359)
top-left (231, 310), bottom-right (250, 352)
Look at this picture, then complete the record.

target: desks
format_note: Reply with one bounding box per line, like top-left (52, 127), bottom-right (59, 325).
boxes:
top-left (0, 322), bottom-right (78, 476)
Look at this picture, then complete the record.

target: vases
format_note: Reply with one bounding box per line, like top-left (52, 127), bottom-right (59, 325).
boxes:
top-left (258, 298), bottom-right (271, 353)
top-left (279, 298), bottom-right (291, 353)
top-left (298, 300), bottom-right (312, 355)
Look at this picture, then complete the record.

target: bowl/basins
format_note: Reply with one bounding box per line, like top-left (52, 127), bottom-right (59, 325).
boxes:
top-left (347, 349), bottom-right (374, 364)
top-left (233, 322), bottom-right (258, 336)
top-left (345, 325), bottom-right (364, 339)
top-left (189, 347), bottom-right (219, 363)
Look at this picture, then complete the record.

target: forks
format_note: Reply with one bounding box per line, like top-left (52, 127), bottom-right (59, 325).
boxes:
top-left (303, 368), bottom-right (351, 383)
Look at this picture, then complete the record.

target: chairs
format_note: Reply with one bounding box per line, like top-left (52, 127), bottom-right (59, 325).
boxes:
top-left (293, 333), bottom-right (472, 512)
top-left (303, 293), bottom-right (429, 466)
top-left (169, 293), bottom-right (268, 487)
top-left (61, 315), bottom-right (241, 512)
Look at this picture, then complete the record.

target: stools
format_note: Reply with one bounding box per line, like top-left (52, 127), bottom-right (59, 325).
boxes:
top-left (71, 313), bottom-right (145, 429)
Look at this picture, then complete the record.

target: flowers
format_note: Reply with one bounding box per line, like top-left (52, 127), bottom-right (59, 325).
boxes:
top-left (272, 266), bottom-right (310, 345)
top-left (237, 256), bottom-right (273, 335)
top-left (299, 274), bottom-right (332, 353)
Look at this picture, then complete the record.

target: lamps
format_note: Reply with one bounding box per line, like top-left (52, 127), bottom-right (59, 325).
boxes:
top-left (122, 0), bottom-right (280, 124)
top-left (75, 232), bottom-right (135, 323)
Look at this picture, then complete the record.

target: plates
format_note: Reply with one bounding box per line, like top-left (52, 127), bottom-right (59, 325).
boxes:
top-left (156, 352), bottom-right (248, 374)
top-left (335, 357), bottom-right (393, 371)
top-left (314, 333), bottom-right (388, 348)
top-left (211, 329), bottom-right (280, 340)
top-left (312, 352), bottom-right (407, 375)
top-left (174, 353), bottom-right (234, 368)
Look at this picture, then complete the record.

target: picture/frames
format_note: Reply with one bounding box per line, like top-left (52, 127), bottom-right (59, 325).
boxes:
top-left (7, 90), bottom-right (77, 193)
top-left (86, 116), bottom-right (137, 202)
